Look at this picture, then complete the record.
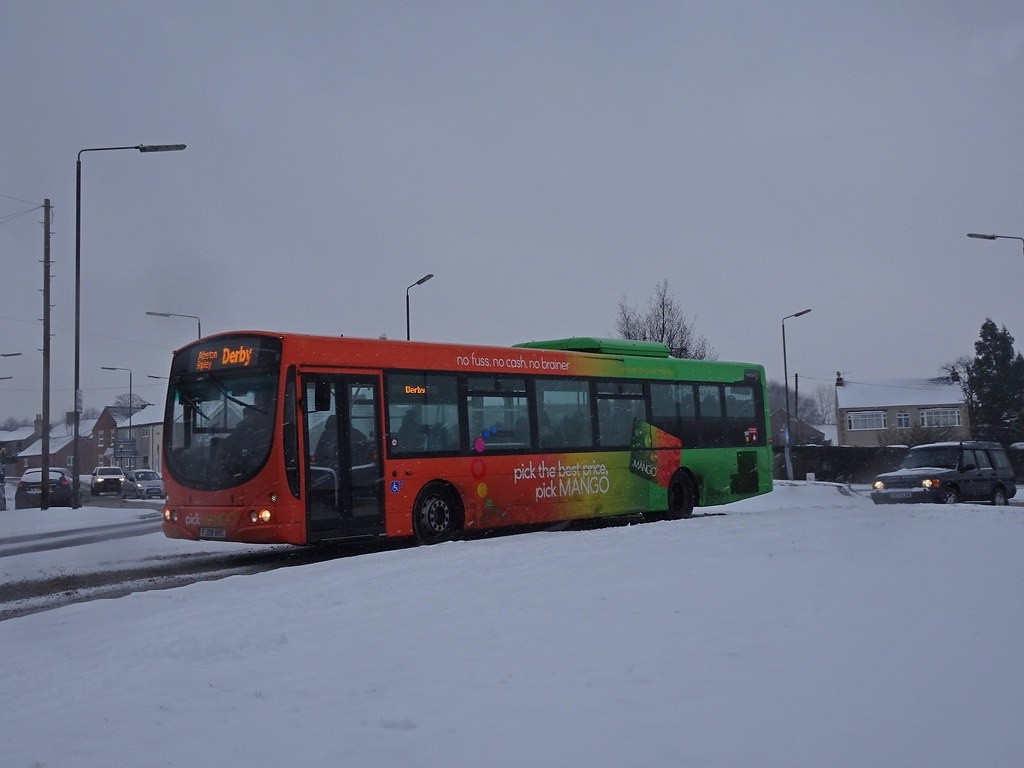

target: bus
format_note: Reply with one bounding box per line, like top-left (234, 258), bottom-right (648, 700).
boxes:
top-left (161, 329), bottom-right (773, 544)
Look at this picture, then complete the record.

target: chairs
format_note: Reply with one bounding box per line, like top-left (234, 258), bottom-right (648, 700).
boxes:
top-left (314, 394), bottom-right (738, 455)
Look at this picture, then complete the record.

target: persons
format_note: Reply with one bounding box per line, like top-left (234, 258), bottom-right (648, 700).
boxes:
top-left (313, 415), bottom-right (367, 460)
top-left (540, 417), bottom-right (563, 448)
top-left (227, 406), bottom-right (269, 471)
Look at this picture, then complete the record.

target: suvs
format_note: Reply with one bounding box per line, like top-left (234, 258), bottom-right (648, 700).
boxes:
top-left (870, 438), bottom-right (1016, 505)
top-left (90, 465), bottom-right (124, 496)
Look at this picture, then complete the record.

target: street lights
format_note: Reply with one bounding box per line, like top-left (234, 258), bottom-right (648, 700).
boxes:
top-left (100, 367), bottom-right (132, 440)
top-left (967, 233), bottom-right (1024, 252)
top-left (406, 274), bottom-right (435, 340)
top-left (119, 445), bottom-right (124, 469)
top-left (73, 143), bottom-right (187, 502)
top-left (781, 309), bottom-right (811, 461)
top-left (146, 311), bottom-right (201, 340)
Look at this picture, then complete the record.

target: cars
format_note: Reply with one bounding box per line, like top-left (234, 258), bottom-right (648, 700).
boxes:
top-left (120, 470), bottom-right (167, 499)
top-left (15, 467), bottom-right (82, 508)
